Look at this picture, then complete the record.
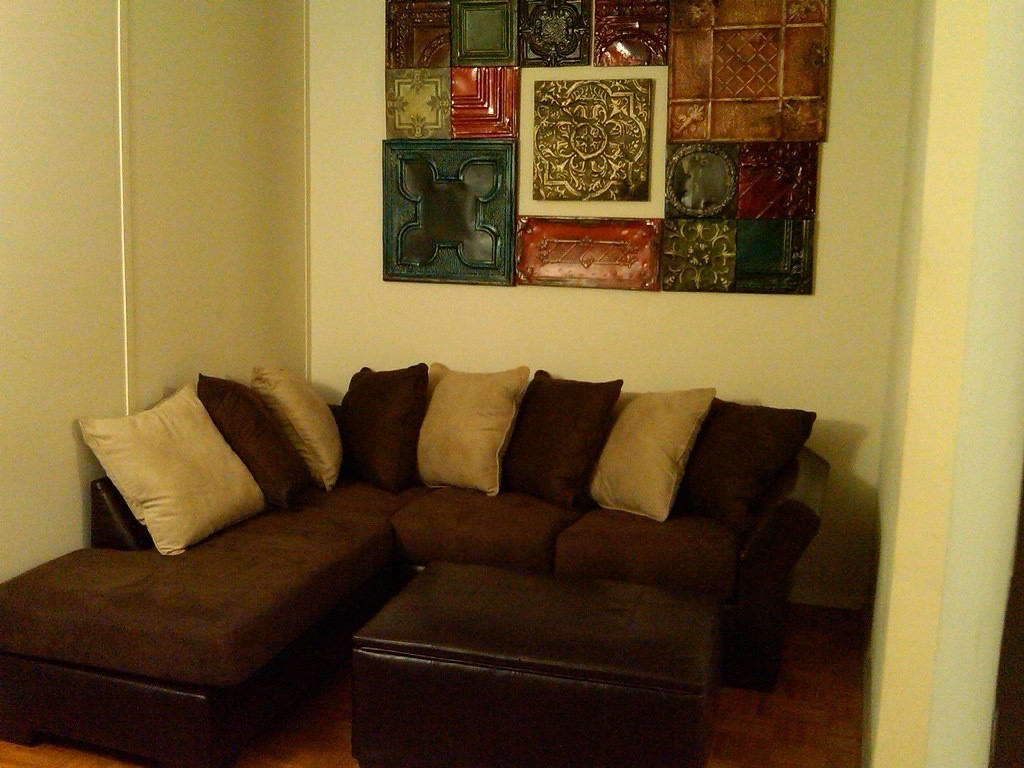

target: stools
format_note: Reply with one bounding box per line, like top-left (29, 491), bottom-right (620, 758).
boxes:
top-left (350, 560), bottom-right (719, 768)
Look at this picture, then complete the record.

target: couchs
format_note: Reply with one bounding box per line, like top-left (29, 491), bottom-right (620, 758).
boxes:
top-left (0, 385), bottom-right (839, 766)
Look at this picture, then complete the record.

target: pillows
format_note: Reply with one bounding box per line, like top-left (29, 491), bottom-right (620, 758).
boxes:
top-left (75, 365), bottom-right (339, 553)
top-left (340, 356), bottom-right (819, 528)
